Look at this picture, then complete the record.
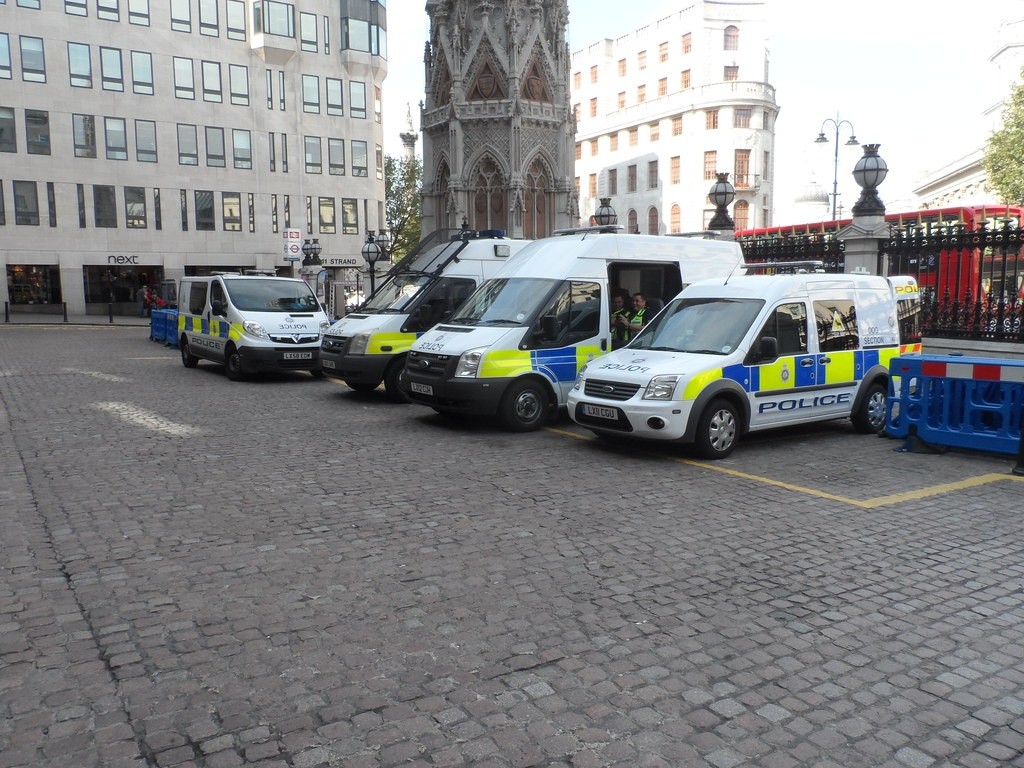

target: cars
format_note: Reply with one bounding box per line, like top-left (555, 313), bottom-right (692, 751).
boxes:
top-left (147, 280), bottom-right (179, 317)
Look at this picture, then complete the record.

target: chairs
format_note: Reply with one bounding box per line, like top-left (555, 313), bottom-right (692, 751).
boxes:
top-left (644, 298), bottom-right (664, 311)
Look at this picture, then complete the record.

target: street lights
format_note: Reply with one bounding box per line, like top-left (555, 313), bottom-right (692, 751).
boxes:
top-left (356, 230), bottom-right (383, 302)
top-left (814, 112), bottom-right (860, 220)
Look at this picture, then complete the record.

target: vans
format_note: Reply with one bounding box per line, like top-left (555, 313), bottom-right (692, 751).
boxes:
top-left (567, 260), bottom-right (922, 460)
top-left (399, 223), bottom-right (749, 433)
top-left (177, 272), bottom-right (332, 380)
top-left (318, 230), bottom-right (542, 402)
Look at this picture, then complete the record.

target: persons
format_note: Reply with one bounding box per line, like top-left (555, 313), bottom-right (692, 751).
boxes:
top-left (135, 285), bottom-right (167, 318)
top-left (609, 292), bottom-right (657, 349)
